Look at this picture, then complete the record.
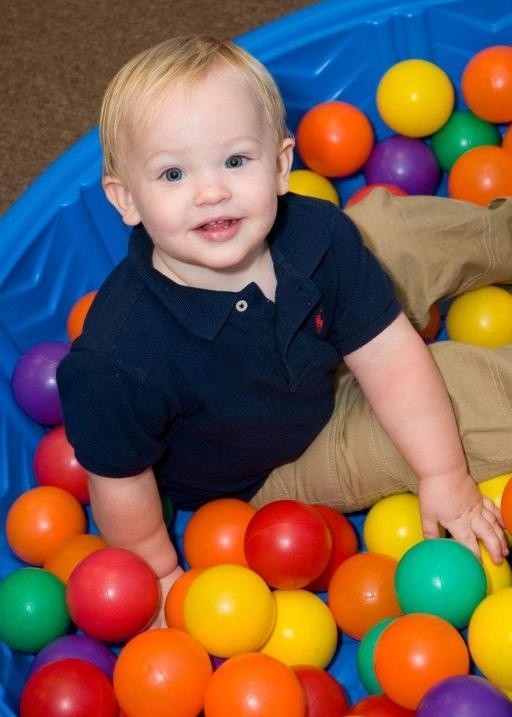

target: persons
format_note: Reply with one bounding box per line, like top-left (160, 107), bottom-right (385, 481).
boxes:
top-left (55, 33), bottom-right (512, 630)
